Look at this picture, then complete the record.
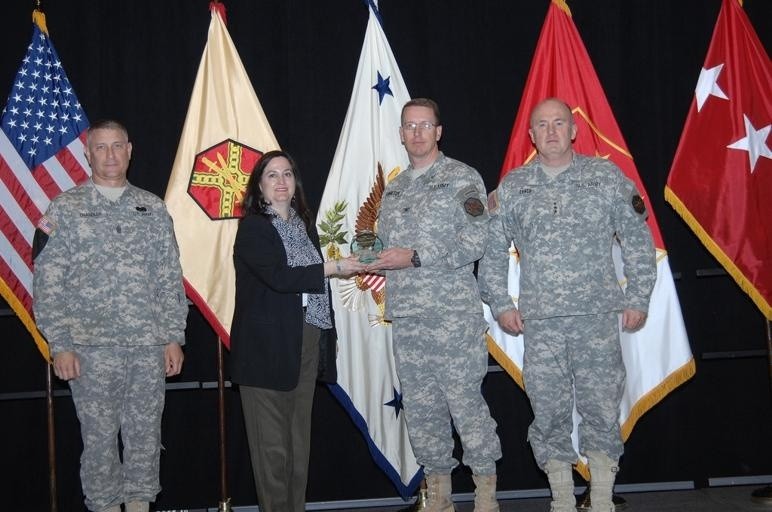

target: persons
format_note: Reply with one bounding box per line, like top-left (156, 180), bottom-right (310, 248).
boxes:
top-left (367, 97), bottom-right (504, 512)
top-left (225, 150), bottom-right (370, 512)
top-left (35, 120), bottom-right (189, 512)
top-left (475, 99), bottom-right (657, 511)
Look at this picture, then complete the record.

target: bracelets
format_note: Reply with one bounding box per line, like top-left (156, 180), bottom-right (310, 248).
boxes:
top-left (335, 258), bottom-right (342, 278)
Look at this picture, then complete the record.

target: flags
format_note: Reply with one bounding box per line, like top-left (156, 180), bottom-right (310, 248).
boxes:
top-left (314, 1), bottom-right (425, 501)
top-left (476, 0), bottom-right (698, 480)
top-left (1, 10), bottom-right (91, 363)
top-left (664, 2), bottom-right (772, 321)
top-left (160, 1), bottom-right (282, 349)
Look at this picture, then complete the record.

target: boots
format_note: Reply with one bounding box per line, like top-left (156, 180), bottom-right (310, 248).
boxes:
top-left (541, 457), bottom-right (579, 512)
top-left (586, 456), bottom-right (622, 512)
top-left (468, 468), bottom-right (503, 512)
top-left (419, 469), bottom-right (457, 512)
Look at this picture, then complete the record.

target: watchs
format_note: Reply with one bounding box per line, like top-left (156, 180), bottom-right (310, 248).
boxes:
top-left (411, 251), bottom-right (421, 268)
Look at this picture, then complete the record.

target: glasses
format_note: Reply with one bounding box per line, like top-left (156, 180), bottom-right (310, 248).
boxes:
top-left (401, 122), bottom-right (440, 130)
